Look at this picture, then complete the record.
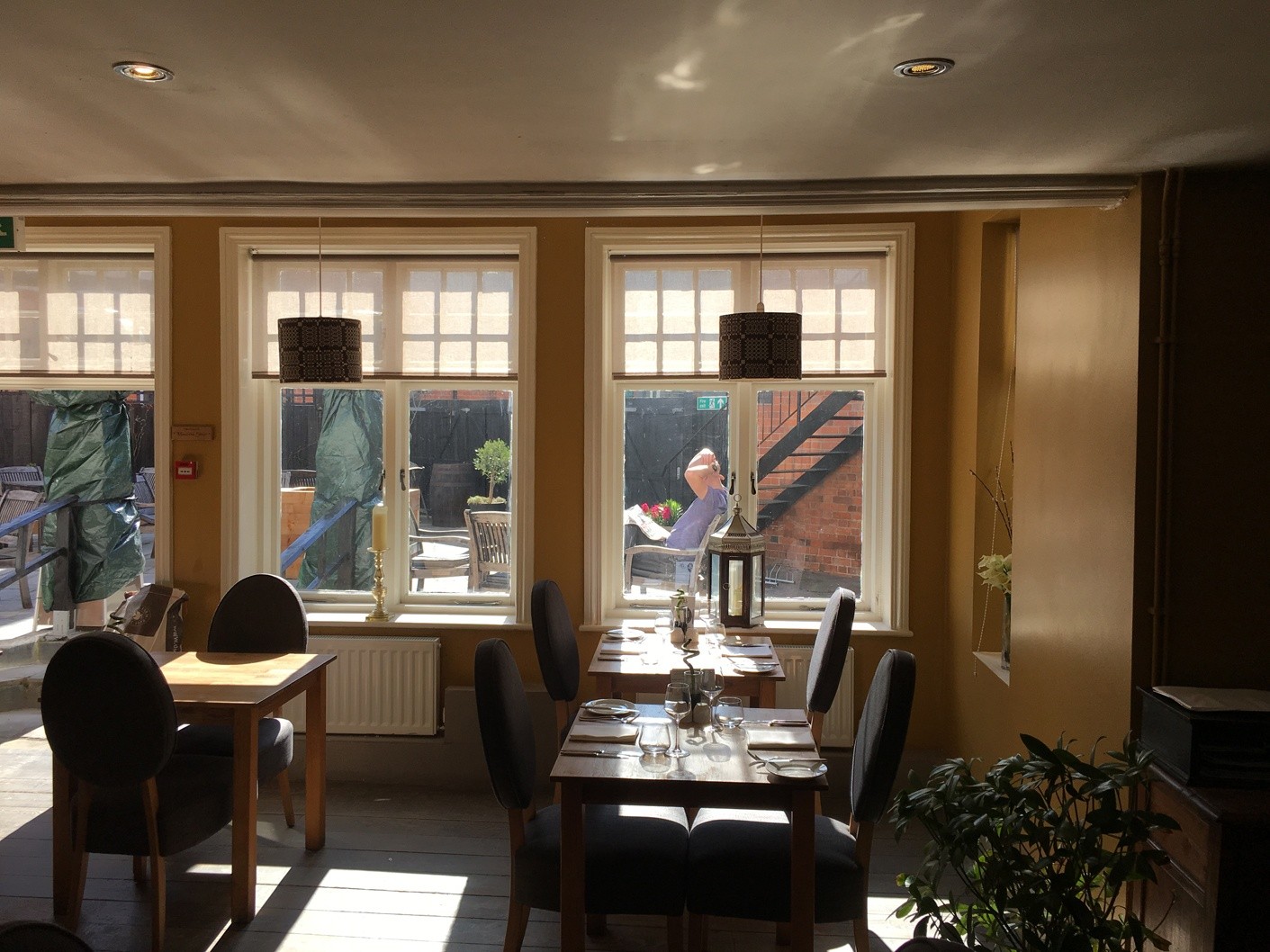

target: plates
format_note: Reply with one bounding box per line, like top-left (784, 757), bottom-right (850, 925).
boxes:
top-left (765, 758), bottom-right (828, 778)
top-left (607, 629), bottom-right (646, 639)
top-left (734, 661), bottom-right (777, 673)
top-left (586, 698), bottom-right (635, 715)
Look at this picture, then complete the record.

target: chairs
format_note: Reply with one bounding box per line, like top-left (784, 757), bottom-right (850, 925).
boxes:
top-left (409, 465), bottom-right (916, 952)
top-left (0, 467), bottom-right (314, 952)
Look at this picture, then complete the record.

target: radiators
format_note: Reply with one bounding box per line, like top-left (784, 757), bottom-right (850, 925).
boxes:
top-left (287, 636), bottom-right (444, 736)
top-left (636, 643), bottom-right (858, 746)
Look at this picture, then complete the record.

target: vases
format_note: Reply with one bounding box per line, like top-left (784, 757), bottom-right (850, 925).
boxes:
top-left (1002, 594), bottom-right (1011, 669)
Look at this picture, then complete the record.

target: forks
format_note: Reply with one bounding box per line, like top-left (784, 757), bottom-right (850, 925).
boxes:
top-left (747, 751), bottom-right (794, 762)
top-left (578, 712), bottom-right (641, 724)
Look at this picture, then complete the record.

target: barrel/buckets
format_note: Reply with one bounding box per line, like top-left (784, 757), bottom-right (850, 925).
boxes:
top-left (428, 463), bottom-right (484, 528)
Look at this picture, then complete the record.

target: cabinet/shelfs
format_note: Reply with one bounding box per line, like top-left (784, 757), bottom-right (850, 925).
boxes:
top-left (1126, 685), bottom-right (1270, 952)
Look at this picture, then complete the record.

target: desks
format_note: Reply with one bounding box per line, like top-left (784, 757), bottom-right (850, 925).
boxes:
top-left (548, 702), bottom-right (830, 952)
top-left (588, 634), bottom-right (785, 708)
top-left (150, 651), bottom-right (337, 927)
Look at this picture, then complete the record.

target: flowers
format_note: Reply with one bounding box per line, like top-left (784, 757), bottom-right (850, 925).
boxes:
top-left (641, 499), bottom-right (684, 525)
top-left (969, 439), bottom-right (1016, 659)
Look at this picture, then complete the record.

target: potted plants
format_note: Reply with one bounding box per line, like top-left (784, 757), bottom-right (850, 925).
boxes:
top-left (468, 439), bottom-right (511, 512)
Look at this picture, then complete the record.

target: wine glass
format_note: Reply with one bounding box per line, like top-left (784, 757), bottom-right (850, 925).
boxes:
top-left (672, 606), bottom-right (692, 655)
top-left (654, 611), bottom-right (675, 645)
top-left (700, 665), bottom-right (725, 732)
top-left (663, 683), bottom-right (692, 758)
top-left (698, 600), bottom-right (717, 644)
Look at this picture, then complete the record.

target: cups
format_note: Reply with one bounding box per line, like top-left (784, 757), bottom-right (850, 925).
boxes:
top-left (639, 633), bottom-right (664, 666)
top-left (715, 697), bottom-right (744, 735)
top-left (706, 623), bottom-right (727, 657)
top-left (684, 670), bottom-right (703, 710)
top-left (639, 722), bottom-right (671, 757)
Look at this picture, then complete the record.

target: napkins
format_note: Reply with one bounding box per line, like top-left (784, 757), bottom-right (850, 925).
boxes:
top-left (600, 643), bottom-right (640, 655)
top-left (569, 724), bottom-right (639, 743)
top-left (748, 730), bottom-right (815, 749)
top-left (721, 646), bottom-right (772, 658)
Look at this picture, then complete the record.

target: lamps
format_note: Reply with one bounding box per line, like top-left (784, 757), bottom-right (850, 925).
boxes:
top-left (278, 215), bottom-right (365, 385)
top-left (709, 494), bottom-right (766, 626)
top-left (719, 213), bottom-right (801, 380)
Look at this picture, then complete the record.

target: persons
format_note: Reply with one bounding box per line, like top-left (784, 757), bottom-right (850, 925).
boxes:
top-left (622, 447), bottom-right (727, 582)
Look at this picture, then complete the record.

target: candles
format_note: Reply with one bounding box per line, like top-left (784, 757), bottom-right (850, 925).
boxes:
top-left (372, 506), bottom-right (388, 549)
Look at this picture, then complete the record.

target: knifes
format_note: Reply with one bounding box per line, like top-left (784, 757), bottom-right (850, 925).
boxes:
top-left (560, 749), bottom-right (643, 758)
top-left (723, 642), bottom-right (771, 648)
top-left (756, 757), bottom-right (828, 763)
top-left (579, 705), bottom-right (640, 712)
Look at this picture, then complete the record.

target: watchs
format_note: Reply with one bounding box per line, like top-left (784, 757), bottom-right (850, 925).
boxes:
top-left (711, 463), bottom-right (717, 473)
